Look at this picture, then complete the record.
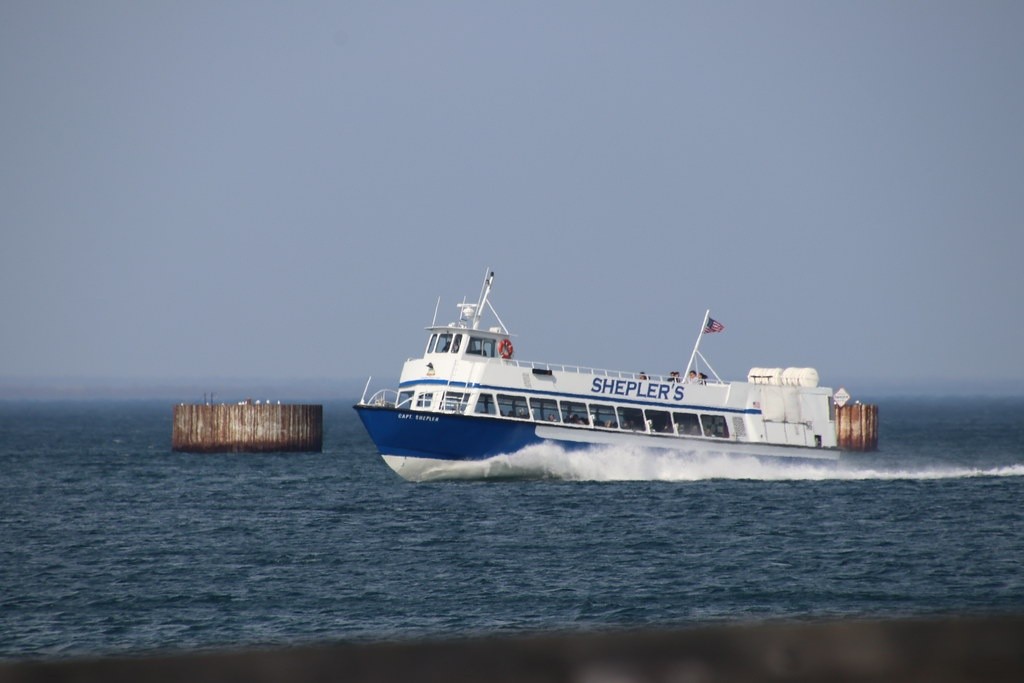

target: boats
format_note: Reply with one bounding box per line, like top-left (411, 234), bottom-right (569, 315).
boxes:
top-left (352, 267), bottom-right (879, 480)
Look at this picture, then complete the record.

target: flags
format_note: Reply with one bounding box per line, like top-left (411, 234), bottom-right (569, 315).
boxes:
top-left (704, 317), bottom-right (725, 334)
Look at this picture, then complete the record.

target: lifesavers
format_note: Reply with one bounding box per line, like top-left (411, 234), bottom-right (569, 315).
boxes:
top-left (498, 338), bottom-right (513, 358)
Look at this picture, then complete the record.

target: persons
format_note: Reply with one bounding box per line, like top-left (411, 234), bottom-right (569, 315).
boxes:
top-left (442, 342), bottom-right (450, 353)
top-left (500, 406), bottom-right (673, 432)
top-left (639, 372), bottom-right (650, 380)
top-left (667, 371), bottom-right (708, 385)
top-left (451, 339), bottom-right (459, 353)
